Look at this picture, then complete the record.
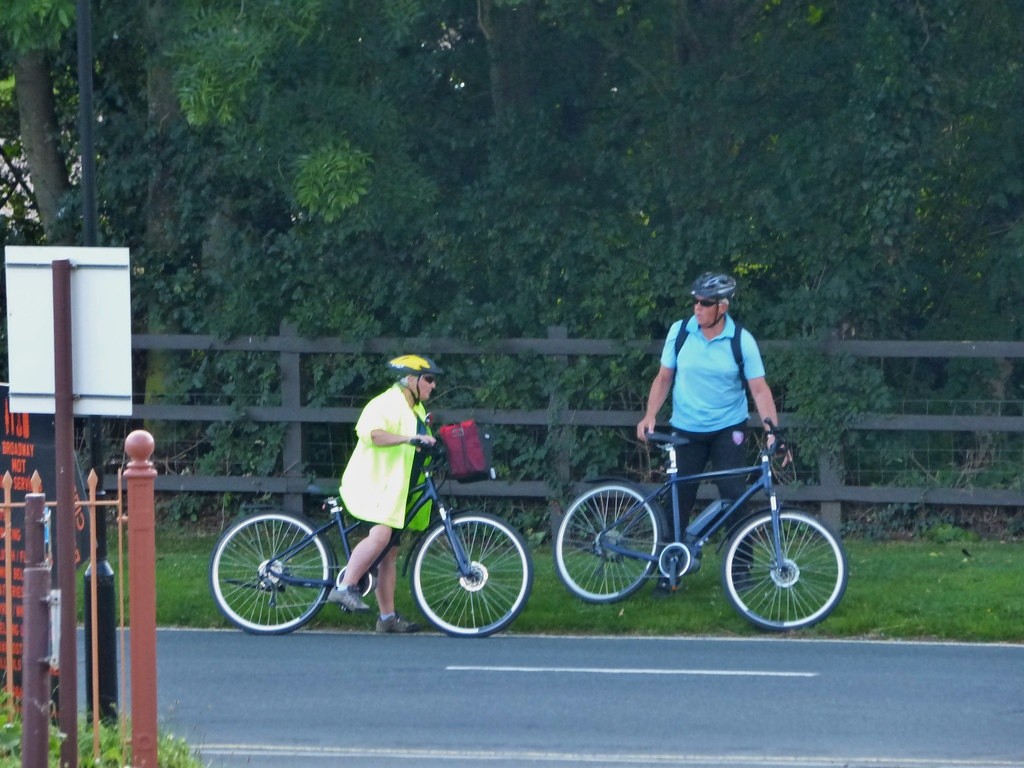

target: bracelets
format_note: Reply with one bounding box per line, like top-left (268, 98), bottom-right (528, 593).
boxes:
top-left (765, 431), bottom-right (771, 439)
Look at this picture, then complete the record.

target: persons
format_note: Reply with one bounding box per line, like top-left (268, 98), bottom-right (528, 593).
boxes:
top-left (637, 272), bottom-right (792, 597)
top-left (328, 354), bottom-right (445, 633)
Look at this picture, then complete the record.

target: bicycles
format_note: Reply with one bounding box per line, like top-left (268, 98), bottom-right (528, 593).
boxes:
top-left (552, 416), bottom-right (850, 634)
top-left (205, 429), bottom-right (535, 640)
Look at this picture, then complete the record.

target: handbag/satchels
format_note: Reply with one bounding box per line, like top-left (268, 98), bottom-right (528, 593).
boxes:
top-left (439, 419), bottom-right (484, 476)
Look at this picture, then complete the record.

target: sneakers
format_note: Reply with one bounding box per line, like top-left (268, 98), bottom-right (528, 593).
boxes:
top-left (328, 585), bottom-right (370, 612)
top-left (376, 615), bottom-right (421, 633)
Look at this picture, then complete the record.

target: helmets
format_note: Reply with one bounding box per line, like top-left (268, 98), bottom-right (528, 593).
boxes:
top-left (689, 272), bottom-right (737, 302)
top-left (385, 355), bottom-right (444, 381)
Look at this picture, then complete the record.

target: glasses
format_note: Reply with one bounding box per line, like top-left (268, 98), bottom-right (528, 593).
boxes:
top-left (693, 299), bottom-right (719, 307)
top-left (424, 376), bottom-right (433, 384)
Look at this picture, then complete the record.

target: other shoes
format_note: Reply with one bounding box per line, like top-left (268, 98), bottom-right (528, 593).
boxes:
top-left (733, 572), bottom-right (752, 589)
top-left (652, 578), bottom-right (672, 599)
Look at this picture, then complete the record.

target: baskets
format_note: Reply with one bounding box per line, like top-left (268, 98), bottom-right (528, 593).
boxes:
top-left (438, 422), bottom-right (492, 484)
